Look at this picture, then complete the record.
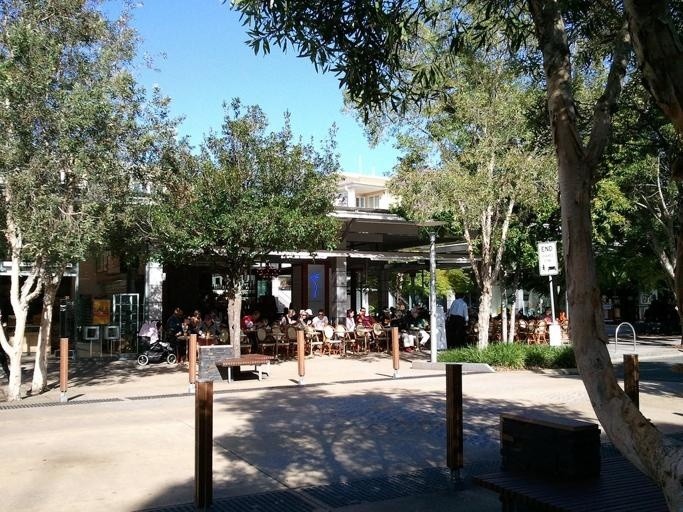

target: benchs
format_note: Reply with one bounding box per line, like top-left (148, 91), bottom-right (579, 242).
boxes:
top-left (498, 411), bottom-right (602, 478)
top-left (215, 353), bottom-right (274, 382)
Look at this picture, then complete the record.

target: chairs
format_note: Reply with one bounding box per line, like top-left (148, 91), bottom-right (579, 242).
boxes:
top-left (179, 316), bottom-right (548, 361)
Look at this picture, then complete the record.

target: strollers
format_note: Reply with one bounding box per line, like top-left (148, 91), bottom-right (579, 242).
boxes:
top-left (137, 320), bottom-right (176, 365)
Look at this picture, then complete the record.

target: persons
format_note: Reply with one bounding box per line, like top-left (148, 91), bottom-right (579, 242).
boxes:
top-left (164, 297), bottom-right (431, 363)
top-left (465, 305), bottom-right (569, 346)
top-left (446, 292), bottom-right (469, 349)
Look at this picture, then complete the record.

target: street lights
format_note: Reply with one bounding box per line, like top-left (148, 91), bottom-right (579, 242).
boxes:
top-left (417, 218), bottom-right (446, 362)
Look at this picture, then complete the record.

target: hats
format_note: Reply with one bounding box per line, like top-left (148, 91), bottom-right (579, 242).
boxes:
top-left (306, 309), bottom-right (313, 316)
top-left (384, 308), bottom-right (391, 312)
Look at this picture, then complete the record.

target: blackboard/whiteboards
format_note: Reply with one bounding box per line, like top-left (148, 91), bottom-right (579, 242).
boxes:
top-left (199, 345), bottom-right (234, 381)
